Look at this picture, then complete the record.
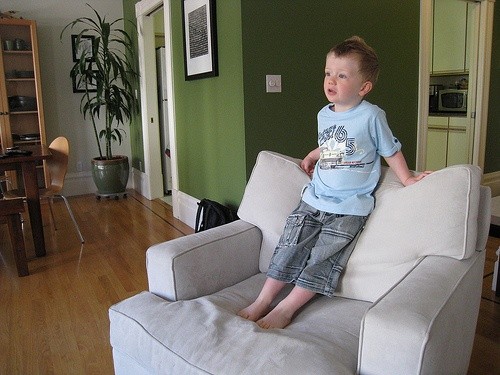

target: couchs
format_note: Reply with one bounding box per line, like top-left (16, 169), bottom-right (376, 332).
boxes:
top-left (107, 149), bottom-right (492, 375)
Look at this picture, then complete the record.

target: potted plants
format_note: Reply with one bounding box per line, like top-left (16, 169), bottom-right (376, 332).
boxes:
top-left (60, 3), bottom-right (140, 194)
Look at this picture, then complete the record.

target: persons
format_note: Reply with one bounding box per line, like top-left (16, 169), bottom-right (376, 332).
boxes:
top-left (238, 36), bottom-right (433, 329)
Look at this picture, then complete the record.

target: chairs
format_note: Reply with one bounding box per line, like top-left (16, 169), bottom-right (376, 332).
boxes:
top-left (38, 136), bottom-right (85, 244)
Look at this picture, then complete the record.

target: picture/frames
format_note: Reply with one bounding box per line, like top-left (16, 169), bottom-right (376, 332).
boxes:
top-left (71, 33), bottom-right (96, 64)
top-left (180, 0), bottom-right (218, 82)
top-left (72, 70), bottom-right (100, 92)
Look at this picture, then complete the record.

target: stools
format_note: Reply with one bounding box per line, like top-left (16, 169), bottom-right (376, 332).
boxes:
top-left (1, 189), bottom-right (30, 279)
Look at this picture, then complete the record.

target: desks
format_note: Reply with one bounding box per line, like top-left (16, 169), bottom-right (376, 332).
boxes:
top-left (0, 145), bottom-right (53, 258)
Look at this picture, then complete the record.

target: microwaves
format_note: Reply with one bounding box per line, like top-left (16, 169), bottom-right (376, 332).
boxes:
top-left (439, 89), bottom-right (468, 112)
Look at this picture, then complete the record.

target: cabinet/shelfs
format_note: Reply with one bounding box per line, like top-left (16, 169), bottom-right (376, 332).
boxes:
top-left (0, 17), bottom-right (53, 206)
top-left (430, 2), bottom-right (470, 76)
top-left (426, 117), bottom-right (468, 172)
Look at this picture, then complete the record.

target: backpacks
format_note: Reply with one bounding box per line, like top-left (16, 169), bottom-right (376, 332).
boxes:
top-left (195, 198), bottom-right (231, 233)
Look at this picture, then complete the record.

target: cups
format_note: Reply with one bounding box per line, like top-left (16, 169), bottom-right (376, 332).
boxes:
top-left (4, 40), bottom-right (14, 50)
